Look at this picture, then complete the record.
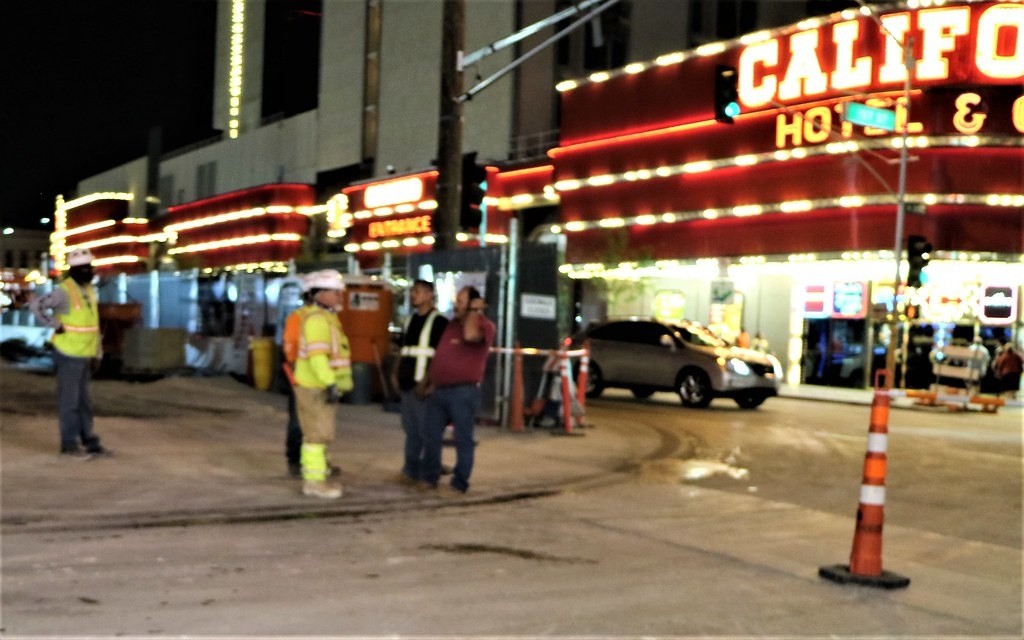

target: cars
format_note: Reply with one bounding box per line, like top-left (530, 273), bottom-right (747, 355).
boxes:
top-left (0, 308), bottom-right (55, 358)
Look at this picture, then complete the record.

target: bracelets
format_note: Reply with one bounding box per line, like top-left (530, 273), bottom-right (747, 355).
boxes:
top-left (468, 306), bottom-right (483, 314)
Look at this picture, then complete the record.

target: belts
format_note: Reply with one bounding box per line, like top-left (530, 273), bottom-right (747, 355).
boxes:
top-left (435, 380), bottom-right (476, 390)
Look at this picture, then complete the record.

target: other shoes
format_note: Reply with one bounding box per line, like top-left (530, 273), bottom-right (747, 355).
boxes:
top-left (302, 481), bottom-right (343, 499)
top-left (406, 482), bottom-right (439, 496)
top-left (383, 471), bottom-right (416, 485)
top-left (289, 463), bottom-right (341, 476)
top-left (441, 484), bottom-right (461, 498)
top-left (58, 444), bottom-right (115, 460)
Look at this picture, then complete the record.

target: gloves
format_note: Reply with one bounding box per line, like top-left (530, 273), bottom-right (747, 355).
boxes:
top-left (55, 323), bottom-right (65, 334)
top-left (324, 384), bottom-right (342, 403)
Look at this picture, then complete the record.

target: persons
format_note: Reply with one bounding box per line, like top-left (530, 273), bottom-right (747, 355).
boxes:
top-left (30, 248), bottom-right (116, 459)
top-left (407, 285), bottom-right (496, 494)
top-left (389, 278), bottom-right (448, 487)
top-left (964, 335), bottom-right (1024, 398)
top-left (282, 268), bottom-right (354, 501)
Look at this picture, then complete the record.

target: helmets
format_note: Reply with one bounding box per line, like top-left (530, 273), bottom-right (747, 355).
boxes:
top-left (68, 248), bottom-right (95, 267)
top-left (301, 269), bottom-right (345, 293)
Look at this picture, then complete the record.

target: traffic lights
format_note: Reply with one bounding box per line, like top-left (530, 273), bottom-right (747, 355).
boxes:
top-left (715, 64), bottom-right (741, 124)
top-left (460, 150), bottom-right (493, 231)
top-left (907, 235), bottom-right (934, 284)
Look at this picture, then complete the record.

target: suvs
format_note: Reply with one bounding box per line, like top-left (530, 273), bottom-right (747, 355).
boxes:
top-left (569, 318), bottom-right (784, 410)
